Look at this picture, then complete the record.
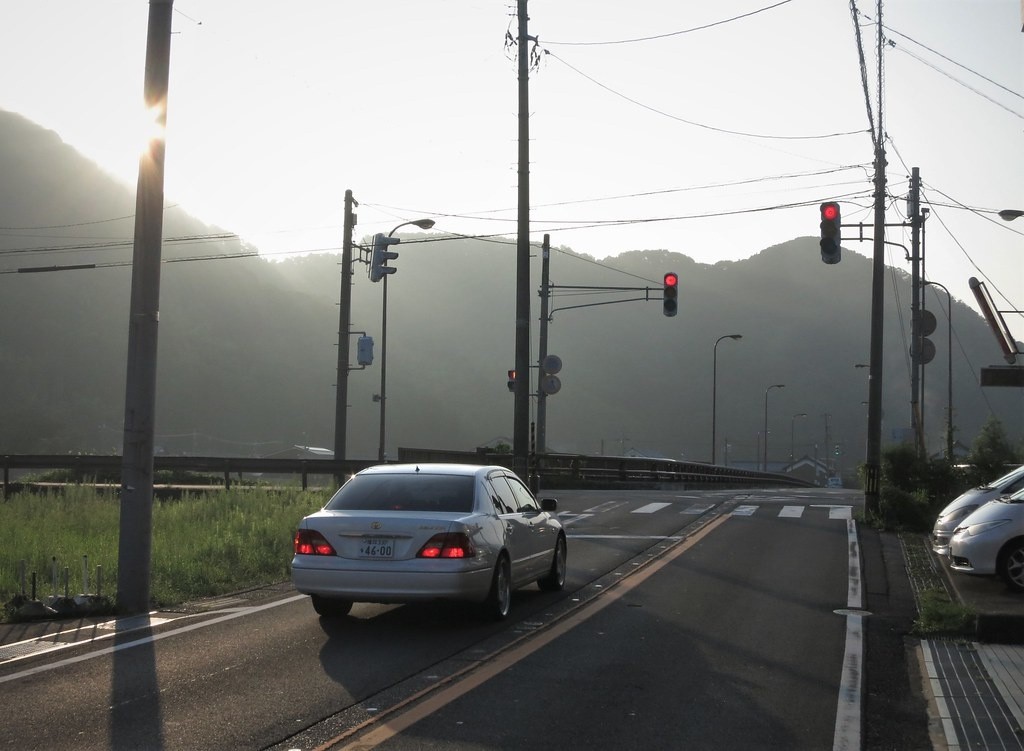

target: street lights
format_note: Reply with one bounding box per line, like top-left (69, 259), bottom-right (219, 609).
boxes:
top-left (756, 428), bottom-right (771, 470)
top-left (712, 332), bottom-right (744, 466)
top-left (791, 413), bottom-right (808, 472)
top-left (378, 217), bottom-right (435, 466)
top-left (763, 383), bottom-right (786, 472)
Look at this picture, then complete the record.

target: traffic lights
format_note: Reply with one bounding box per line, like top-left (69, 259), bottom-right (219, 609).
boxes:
top-left (508, 368), bottom-right (516, 391)
top-left (663, 273), bottom-right (678, 317)
top-left (369, 234), bottom-right (401, 281)
top-left (819, 201), bottom-right (842, 264)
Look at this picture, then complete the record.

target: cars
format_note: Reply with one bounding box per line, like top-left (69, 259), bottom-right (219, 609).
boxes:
top-left (931, 467), bottom-right (1024, 556)
top-left (946, 488), bottom-right (1023, 595)
top-left (292, 463), bottom-right (568, 623)
